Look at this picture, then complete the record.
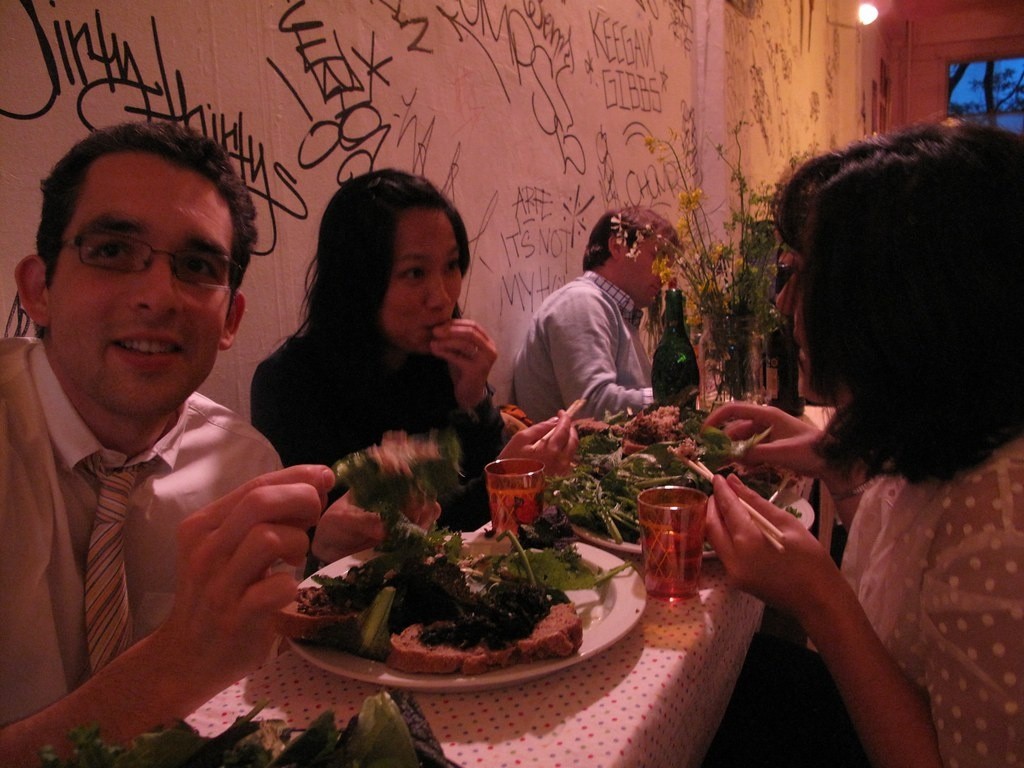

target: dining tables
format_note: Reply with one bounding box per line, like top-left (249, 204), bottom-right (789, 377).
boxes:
top-left (180, 405), bottom-right (836, 768)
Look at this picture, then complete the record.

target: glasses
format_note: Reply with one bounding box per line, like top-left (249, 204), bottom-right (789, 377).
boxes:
top-left (50, 229), bottom-right (245, 287)
top-left (774, 262), bottom-right (799, 288)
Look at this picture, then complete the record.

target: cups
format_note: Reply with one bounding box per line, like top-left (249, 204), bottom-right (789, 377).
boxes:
top-left (638, 487), bottom-right (709, 594)
top-left (486, 460), bottom-right (546, 535)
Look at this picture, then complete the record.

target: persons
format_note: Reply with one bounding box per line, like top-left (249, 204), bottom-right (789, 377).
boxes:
top-left (0, 118), bottom-right (336, 768)
top-left (249, 167), bottom-right (579, 532)
top-left (512, 204), bottom-right (679, 424)
top-left (701, 108), bottom-right (1023, 768)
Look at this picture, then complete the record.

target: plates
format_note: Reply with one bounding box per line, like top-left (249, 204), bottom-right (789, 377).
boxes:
top-left (572, 488), bottom-right (815, 559)
top-left (283, 532), bottom-right (648, 692)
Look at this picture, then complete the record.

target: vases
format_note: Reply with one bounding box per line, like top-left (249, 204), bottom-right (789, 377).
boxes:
top-left (688, 319), bottom-right (770, 414)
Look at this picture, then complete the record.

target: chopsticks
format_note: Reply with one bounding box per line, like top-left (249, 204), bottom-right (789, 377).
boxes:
top-left (532, 397), bottom-right (589, 454)
top-left (668, 443), bottom-right (786, 555)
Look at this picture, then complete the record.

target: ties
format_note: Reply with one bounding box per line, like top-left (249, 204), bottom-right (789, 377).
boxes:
top-left (80, 450), bottom-right (162, 675)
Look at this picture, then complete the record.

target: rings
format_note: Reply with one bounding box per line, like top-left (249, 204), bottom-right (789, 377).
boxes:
top-left (468, 345), bottom-right (479, 359)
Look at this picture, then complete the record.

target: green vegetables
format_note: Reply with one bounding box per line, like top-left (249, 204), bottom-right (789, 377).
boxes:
top-left (38, 411), bottom-right (773, 768)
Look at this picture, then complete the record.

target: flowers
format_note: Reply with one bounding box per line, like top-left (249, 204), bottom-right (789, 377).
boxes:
top-left (608, 114), bottom-right (810, 412)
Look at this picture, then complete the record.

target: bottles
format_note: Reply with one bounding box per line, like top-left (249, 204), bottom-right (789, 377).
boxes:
top-left (766, 265), bottom-right (806, 416)
top-left (650, 290), bottom-right (701, 409)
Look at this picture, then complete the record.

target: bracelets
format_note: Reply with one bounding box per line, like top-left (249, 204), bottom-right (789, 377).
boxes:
top-left (831, 473), bottom-right (881, 501)
top-left (482, 459), bottom-right (508, 489)
top-left (304, 551), bottom-right (330, 580)
